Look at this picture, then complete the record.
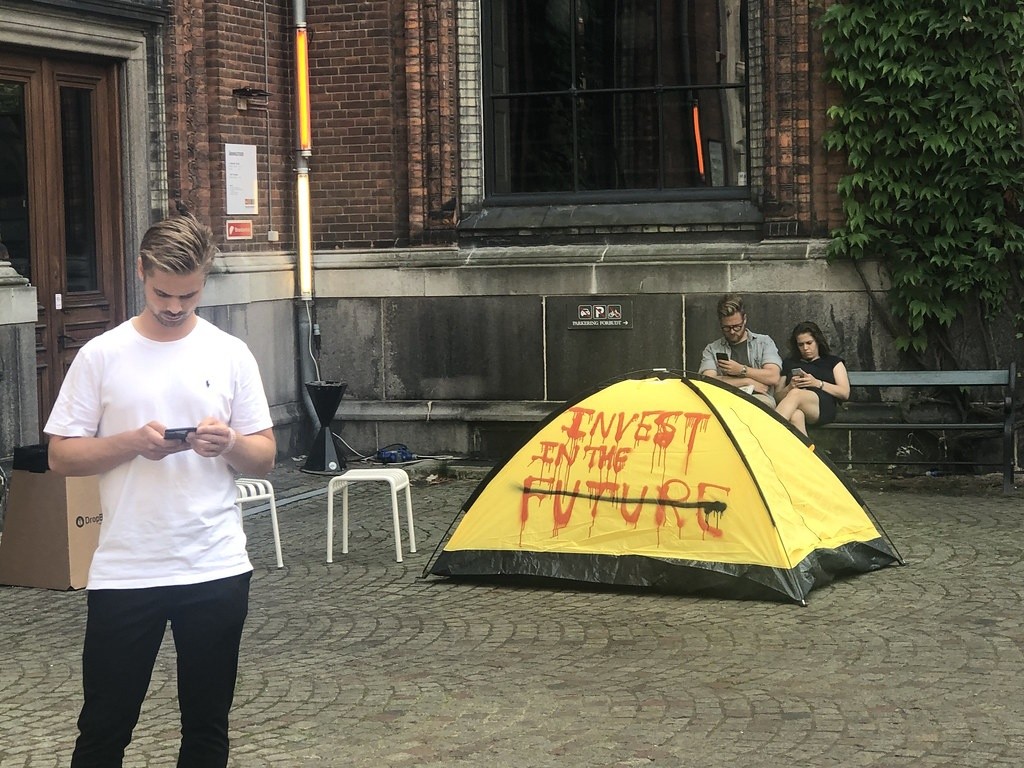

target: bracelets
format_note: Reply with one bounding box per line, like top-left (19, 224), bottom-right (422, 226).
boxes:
top-left (221, 428), bottom-right (236, 454)
top-left (820, 381), bottom-right (823, 389)
top-left (740, 366), bottom-right (747, 377)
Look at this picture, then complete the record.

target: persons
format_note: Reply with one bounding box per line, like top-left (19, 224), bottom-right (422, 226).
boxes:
top-left (698, 295), bottom-right (782, 409)
top-left (43, 216), bottom-right (277, 768)
top-left (774, 321), bottom-right (850, 437)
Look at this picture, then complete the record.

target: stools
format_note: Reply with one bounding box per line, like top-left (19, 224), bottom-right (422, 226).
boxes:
top-left (326, 468), bottom-right (416, 563)
top-left (235, 477), bottom-right (283, 569)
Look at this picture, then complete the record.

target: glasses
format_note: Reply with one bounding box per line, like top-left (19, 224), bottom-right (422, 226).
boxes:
top-left (721, 319), bottom-right (744, 332)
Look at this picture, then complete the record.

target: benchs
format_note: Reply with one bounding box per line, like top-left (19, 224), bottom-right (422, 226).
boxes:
top-left (806, 363), bottom-right (1018, 497)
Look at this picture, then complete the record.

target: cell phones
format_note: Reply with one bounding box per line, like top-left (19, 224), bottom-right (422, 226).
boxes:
top-left (716, 352), bottom-right (728, 370)
top-left (163, 427), bottom-right (197, 445)
top-left (791, 368), bottom-right (805, 383)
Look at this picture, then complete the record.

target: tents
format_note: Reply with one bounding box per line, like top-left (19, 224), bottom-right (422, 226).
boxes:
top-left (423, 368), bottom-right (906, 609)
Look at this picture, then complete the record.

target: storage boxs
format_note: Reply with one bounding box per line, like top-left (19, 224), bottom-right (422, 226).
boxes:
top-left (0, 468), bottom-right (103, 591)
